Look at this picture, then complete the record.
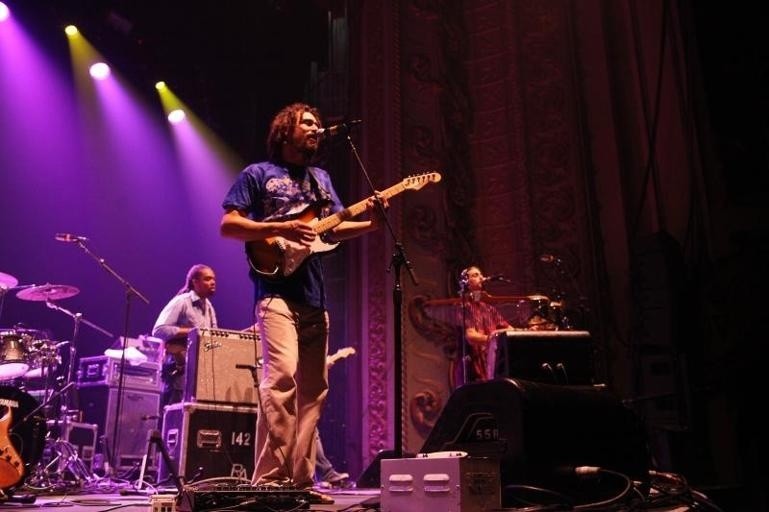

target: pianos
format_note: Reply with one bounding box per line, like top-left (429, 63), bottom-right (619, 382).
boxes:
top-left (495, 329), bottom-right (591, 341)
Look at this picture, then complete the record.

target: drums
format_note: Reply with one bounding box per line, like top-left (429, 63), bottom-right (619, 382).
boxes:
top-left (0, 329), bottom-right (32, 379)
top-left (0, 384), bottom-right (48, 496)
top-left (33, 341), bottom-right (62, 377)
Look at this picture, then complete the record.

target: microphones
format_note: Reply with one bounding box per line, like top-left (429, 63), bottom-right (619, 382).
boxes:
top-left (54, 232), bottom-right (84, 244)
top-left (481, 275), bottom-right (499, 286)
top-left (316, 122), bottom-right (350, 141)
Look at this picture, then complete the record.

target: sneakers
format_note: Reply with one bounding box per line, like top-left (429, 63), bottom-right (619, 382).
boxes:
top-left (321, 471), bottom-right (350, 486)
top-left (301, 488), bottom-right (335, 505)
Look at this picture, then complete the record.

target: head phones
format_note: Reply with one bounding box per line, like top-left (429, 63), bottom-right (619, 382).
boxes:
top-left (457, 266), bottom-right (473, 288)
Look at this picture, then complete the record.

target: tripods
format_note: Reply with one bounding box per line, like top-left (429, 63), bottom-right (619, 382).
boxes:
top-left (41, 322), bottom-right (93, 481)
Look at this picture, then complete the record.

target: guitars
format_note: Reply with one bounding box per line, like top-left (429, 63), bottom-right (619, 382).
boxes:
top-left (244, 171), bottom-right (442, 279)
top-left (2, 400), bottom-right (23, 490)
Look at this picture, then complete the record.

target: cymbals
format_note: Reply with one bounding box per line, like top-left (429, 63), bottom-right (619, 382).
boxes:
top-left (14, 284), bottom-right (80, 302)
top-left (0, 272), bottom-right (20, 295)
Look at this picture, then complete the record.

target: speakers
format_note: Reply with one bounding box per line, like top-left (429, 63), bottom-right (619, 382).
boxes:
top-left (419, 377), bottom-right (651, 507)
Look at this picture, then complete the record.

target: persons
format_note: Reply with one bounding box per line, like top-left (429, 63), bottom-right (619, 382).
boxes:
top-left (448, 266), bottom-right (538, 390)
top-left (219, 102), bottom-right (390, 504)
top-left (152, 264), bottom-right (218, 451)
top-left (316, 355), bottom-right (349, 483)
